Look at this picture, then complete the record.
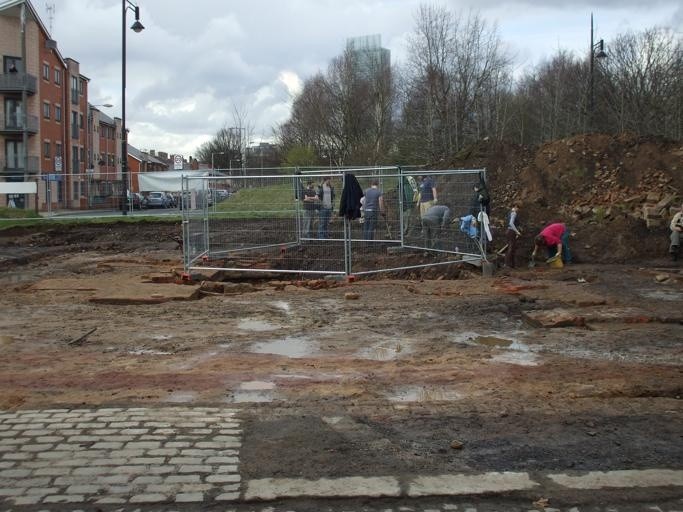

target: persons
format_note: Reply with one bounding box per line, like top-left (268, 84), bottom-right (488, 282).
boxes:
top-left (302, 175), bottom-right (573, 267)
top-left (669, 204), bottom-right (683, 261)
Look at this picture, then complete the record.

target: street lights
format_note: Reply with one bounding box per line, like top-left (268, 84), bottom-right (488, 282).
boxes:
top-left (588, 12), bottom-right (607, 131)
top-left (229, 160), bottom-right (240, 176)
top-left (87, 103), bottom-right (113, 207)
top-left (121, 0), bottom-right (143, 215)
top-left (211, 152), bottom-right (223, 188)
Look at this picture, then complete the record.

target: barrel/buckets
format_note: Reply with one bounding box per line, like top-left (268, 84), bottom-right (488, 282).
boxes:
top-left (547, 255), bottom-right (564, 269)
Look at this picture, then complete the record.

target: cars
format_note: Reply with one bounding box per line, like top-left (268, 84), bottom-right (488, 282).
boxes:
top-left (119, 188), bottom-right (233, 210)
